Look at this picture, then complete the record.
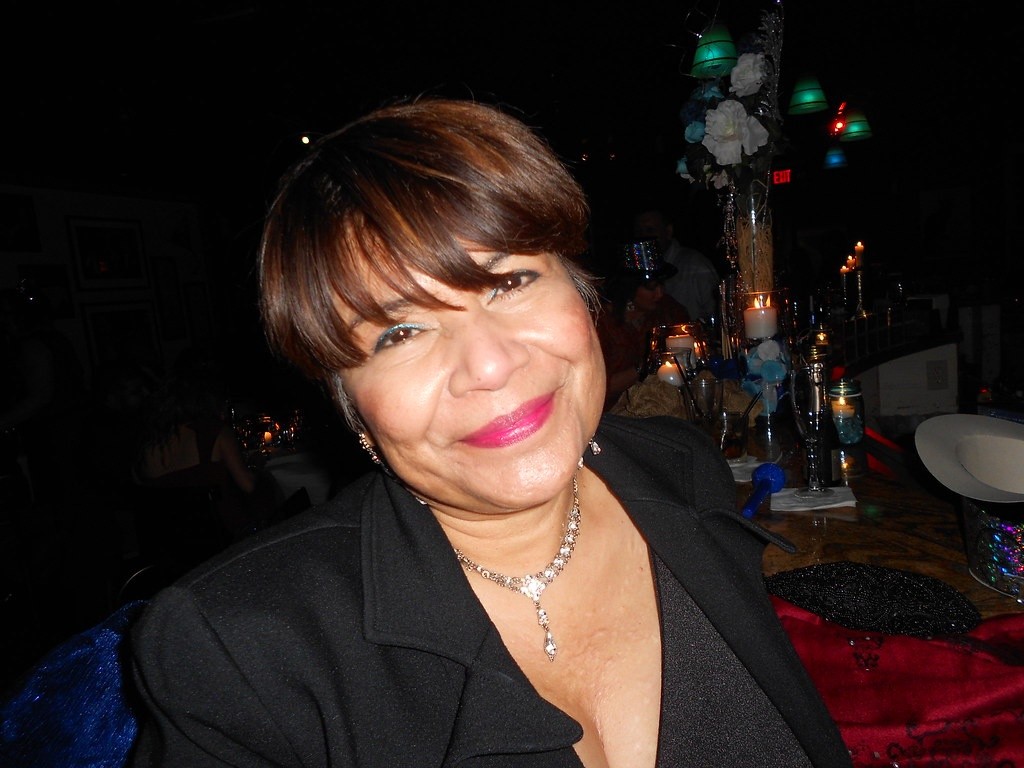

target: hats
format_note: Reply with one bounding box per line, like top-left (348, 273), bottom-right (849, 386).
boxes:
top-left (913, 413), bottom-right (1023, 503)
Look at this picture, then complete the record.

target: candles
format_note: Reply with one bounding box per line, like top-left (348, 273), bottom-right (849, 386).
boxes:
top-left (855, 241), bottom-right (864, 266)
top-left (657, 360), bottom-right (684, 388)
top-left (840, 265), bottom-right (849, 273)
top-left (743, 295), bottom-right (777, 339)
top-left (847, 255), bottom-right (855, 267)
top-left (666, 336), bottom-right (697, 370)
top-left (832, 397), bottom-right (855, 419)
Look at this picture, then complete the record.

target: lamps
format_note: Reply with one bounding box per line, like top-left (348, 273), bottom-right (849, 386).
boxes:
top-left (840, 113), bottom-right (871, 142)
top-left (691, 28), bottom-right (738, 77)
top-left (789, 80), bottom-right (827, 114)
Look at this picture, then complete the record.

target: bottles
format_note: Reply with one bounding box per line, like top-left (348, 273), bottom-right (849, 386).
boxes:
top-left (806, 294), bottom-right (817, 326)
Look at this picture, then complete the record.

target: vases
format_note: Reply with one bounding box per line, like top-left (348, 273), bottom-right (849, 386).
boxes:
top-left (733, 210), bottom-right (773, 306)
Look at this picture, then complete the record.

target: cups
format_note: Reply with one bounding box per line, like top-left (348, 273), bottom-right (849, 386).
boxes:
top-left (703, 411), bottom-right (749, 464)
top-left (683, 379), bottom-right (724, 428)
top-left (828, 379), bottom-right (865, 446)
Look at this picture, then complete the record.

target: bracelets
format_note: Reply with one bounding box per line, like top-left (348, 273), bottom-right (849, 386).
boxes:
top-left (635, 365), bottom-right (642, 377)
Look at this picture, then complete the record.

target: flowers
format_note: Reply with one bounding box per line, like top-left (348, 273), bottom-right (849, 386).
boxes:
top-left (676, 51), bottom-right (780, 209)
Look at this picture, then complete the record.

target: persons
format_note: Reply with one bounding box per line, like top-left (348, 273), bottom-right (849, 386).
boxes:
top-left (593, 208), bottom-right (720, 412)
top-left (123, 99), bottom-right (853, 768)
top-left (134, 368), bottom-right (256, 592)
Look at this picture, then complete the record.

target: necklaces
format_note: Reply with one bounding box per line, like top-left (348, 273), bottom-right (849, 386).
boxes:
top-left (452, 472), bottom-right (581, 661)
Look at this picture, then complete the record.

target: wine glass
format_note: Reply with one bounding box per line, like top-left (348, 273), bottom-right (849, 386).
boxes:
top-left (788, 365), bottom-right (836, 500)
